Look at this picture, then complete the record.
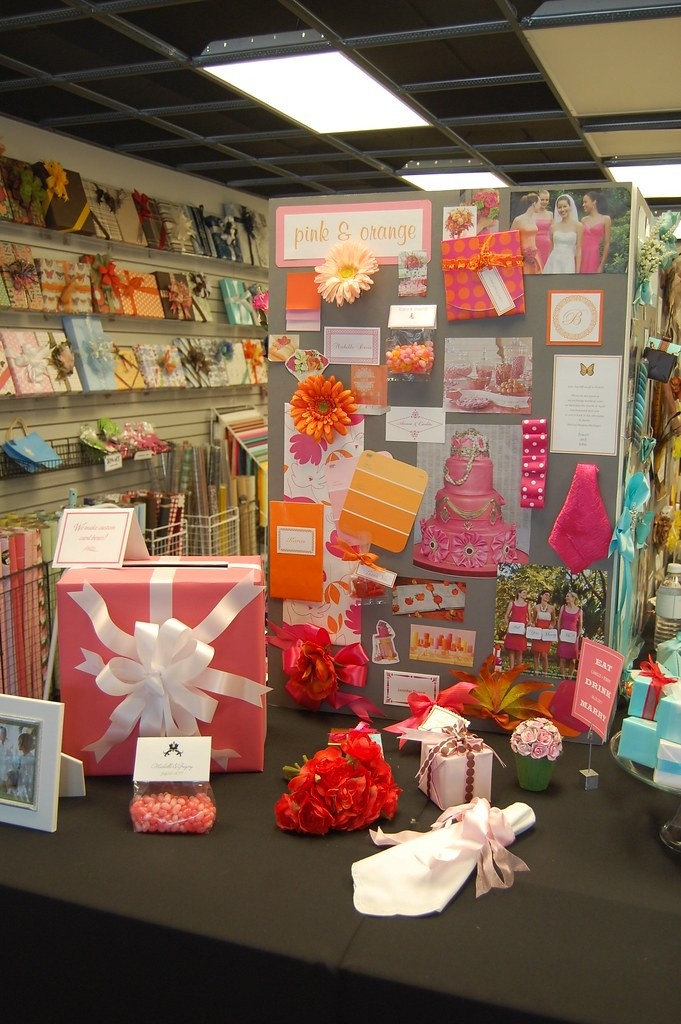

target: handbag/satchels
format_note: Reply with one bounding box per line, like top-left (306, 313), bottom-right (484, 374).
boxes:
top-left (1, 417), bottom-right (63, 473)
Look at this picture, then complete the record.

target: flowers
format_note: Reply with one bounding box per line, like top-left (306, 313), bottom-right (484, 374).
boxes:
top-left (274, 731), bottom-right (404, 834)
top-left (472, 189), bottom-right (500, 220)
top-left (290, 374), bottom-right (358, 443)
top-left (509, 717), bottom-right (562, 761)
top-left (445, 208), bottom-right (475, 239)
top-left (294, 641), bottom-right (346, 700)
top-left (637, 215), bottom-right (678, 284)
top-left (522, 248), bottom-right (538, 264)
top-left (314, 241), bottom-right (379, 308)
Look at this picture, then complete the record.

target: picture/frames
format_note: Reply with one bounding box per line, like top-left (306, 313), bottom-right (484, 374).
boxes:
top-left (0, 693), bottom-right (65, 832)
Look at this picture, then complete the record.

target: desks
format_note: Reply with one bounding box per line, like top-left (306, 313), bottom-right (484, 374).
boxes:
top-left (1, 703), bottom-right (681, 1024)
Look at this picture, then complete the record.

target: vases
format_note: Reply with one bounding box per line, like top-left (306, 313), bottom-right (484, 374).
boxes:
top-left (515, 753), bottom-right (554, 791)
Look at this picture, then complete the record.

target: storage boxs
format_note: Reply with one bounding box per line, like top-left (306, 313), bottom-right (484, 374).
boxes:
top-left (396, 725), bottom-right (506, 811)
top-left (0, 315), bottom-right (268, 398)
top-left (56, 557), bottom-right (268, 777)
top-left (329, 721), bottom-right (385, 761)
top-left (0, 242), bottom-right (270, 325)
top-left (616, 630), bottom-right (681, 789)
top-left (0, 155), bottom-right (269, 269)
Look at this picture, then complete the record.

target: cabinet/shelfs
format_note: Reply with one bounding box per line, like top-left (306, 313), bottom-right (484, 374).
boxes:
top-left (0, 220), bottom-right (270, 400)
